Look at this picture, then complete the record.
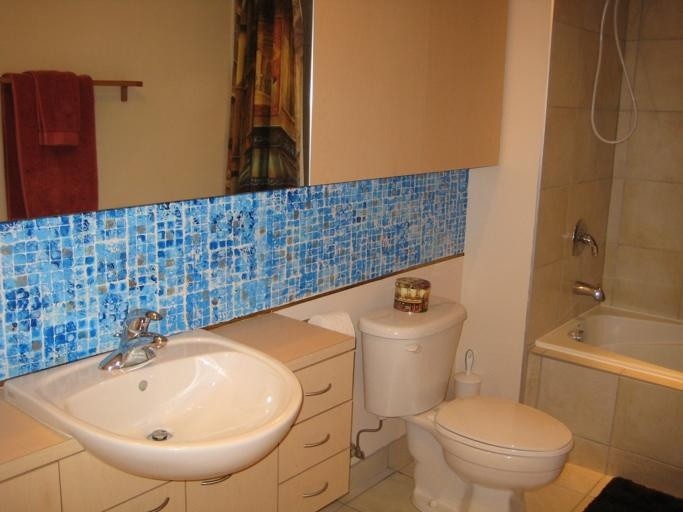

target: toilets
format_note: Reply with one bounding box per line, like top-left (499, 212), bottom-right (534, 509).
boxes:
top-left (358, 295), bottom-right (574, 512)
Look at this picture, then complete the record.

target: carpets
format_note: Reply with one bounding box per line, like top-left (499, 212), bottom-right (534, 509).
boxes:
top-left (579, 472), bottom-right (680, 512)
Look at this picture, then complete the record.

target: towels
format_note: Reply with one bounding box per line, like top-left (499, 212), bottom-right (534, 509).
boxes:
top-left (0, 64), bottom-right (100, 225)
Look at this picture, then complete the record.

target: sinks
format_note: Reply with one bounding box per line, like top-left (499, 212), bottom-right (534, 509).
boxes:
top-left (5, 329), bottom-right (303, 481)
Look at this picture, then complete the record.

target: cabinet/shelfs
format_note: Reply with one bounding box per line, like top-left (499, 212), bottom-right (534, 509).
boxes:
top-left (186, 436), bottom-right (278, 511)
top-left (207, 303), bottom-right (359, 512)
top-left (2, 459), bottom-right (60, 512)
top-left (55, 452), bottom-right (182, 512)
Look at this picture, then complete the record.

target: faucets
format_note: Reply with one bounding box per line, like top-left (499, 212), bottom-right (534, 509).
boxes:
top-left (99, 309), bottom-right (167, 370)
top-left (574, 281), bottom-right (606, 302)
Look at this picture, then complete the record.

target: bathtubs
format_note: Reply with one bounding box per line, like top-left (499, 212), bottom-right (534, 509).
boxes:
top-left (523, 304), bottom-right (682, 499)
